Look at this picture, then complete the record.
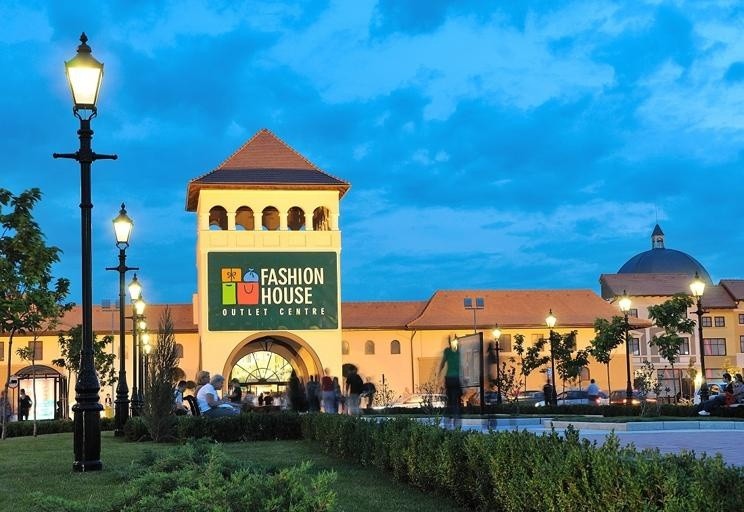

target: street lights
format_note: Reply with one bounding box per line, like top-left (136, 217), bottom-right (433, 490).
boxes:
top-left (52, 28), bottom-right (121, 472)
top-left (137, 298), bottom-right (153, 416)
top-left (124, 271), bottom-right (147, 420)
top-left (463, 297), bottom-right (485, 334)
top-left (688, 270), bottom-right (710, 402)
top-left (545, 306), bottom-right (558, 406)
top-left (489, 321), bottom-right (505, 405)
top-left (105, 197), bottom-right (140, 438)
top-left (101, 299), bottom-right (121, 417)
top-left (617, 285), bottom-right (635, 407)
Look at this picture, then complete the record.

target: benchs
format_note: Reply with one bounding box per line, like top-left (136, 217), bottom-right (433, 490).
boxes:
top-left (181, 394), bottom-right (201, 416)
top-left (719, 399), bottom-right (743, 416)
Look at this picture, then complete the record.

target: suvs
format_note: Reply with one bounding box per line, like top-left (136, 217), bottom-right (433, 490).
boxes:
top-left (374, 391), bottom-right (447, 410)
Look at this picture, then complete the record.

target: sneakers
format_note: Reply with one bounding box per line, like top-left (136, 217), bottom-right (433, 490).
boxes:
top-left (698, 410), bottom-right (711, 416)
top-left (725, 392), bottom-right (736, 405)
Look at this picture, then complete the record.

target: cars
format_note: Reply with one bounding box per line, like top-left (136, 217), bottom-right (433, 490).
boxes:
top-left (468, 390), bottom-right (510, 406)
top-left (693, 381), bottom-right (728, 405)
top-left (511, 388), bottom-right (645, 408)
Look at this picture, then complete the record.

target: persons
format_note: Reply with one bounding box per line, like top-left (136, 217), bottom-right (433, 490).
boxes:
top-left (0, 390), bottom-right (11, 424)
top-left (697, 373), bottom-right (734, 415)
top-left (701, 377), bottom-right (709, 403)
top-left (56, 401), bottom-right (61, 419)
top-left (720, 373), bottom-right (744, 404)
top-left (174, 371), bottom-right (289, 417)
top-left (106, 393), bottom-right (111, 406)
top-left (486, 383), bottom-right (498, 431)
top-left (586, 379), bottom-right (598, 406)
top-left (438, 334), bottom-right (462, 430)
top-left (300, 366), bottom-right (376, 414)
top-left (18, 389), bottom-right (32, 421)
top-left (543, 379), bottom-right (552, 405)
top-left (100, 389), bottom-right (104, 406)
